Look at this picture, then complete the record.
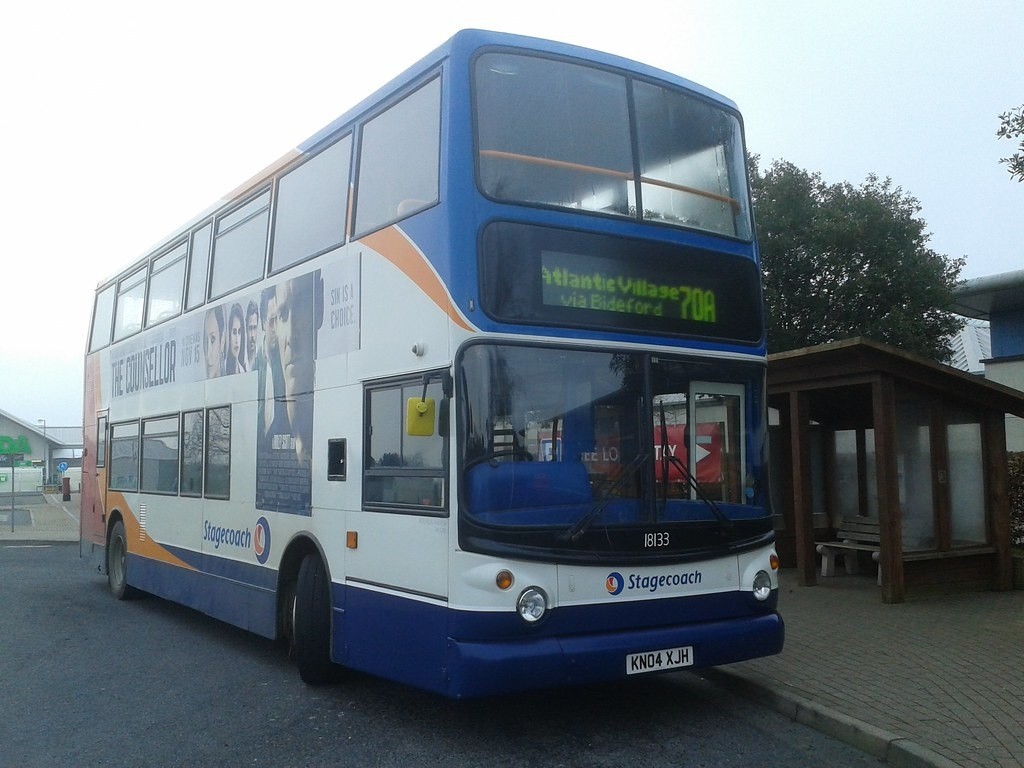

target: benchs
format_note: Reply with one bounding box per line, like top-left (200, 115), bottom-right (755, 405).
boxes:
top-left (814, 514), bottom-right (925, 586)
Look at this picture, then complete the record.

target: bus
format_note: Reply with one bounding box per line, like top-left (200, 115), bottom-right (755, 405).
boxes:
top-left (76, 27), bottom-right (787, 705)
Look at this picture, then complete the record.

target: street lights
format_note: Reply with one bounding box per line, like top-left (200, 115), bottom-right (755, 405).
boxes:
top-left (37, 418), bottom-right (46, 494)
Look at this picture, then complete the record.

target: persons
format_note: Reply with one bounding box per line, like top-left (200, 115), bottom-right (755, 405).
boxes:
top-left (203, 269), bottom-right (325, 516)
